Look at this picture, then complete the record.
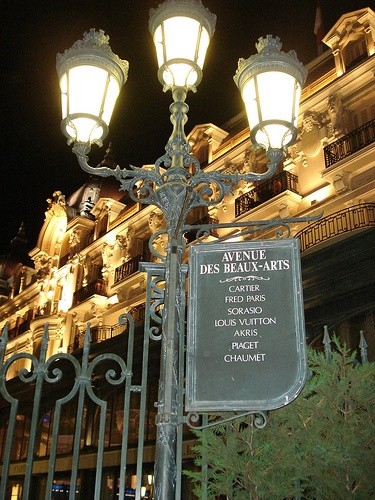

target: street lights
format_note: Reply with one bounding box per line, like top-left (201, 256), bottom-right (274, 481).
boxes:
top-left (49, 3), bottom-right (305, 497)
